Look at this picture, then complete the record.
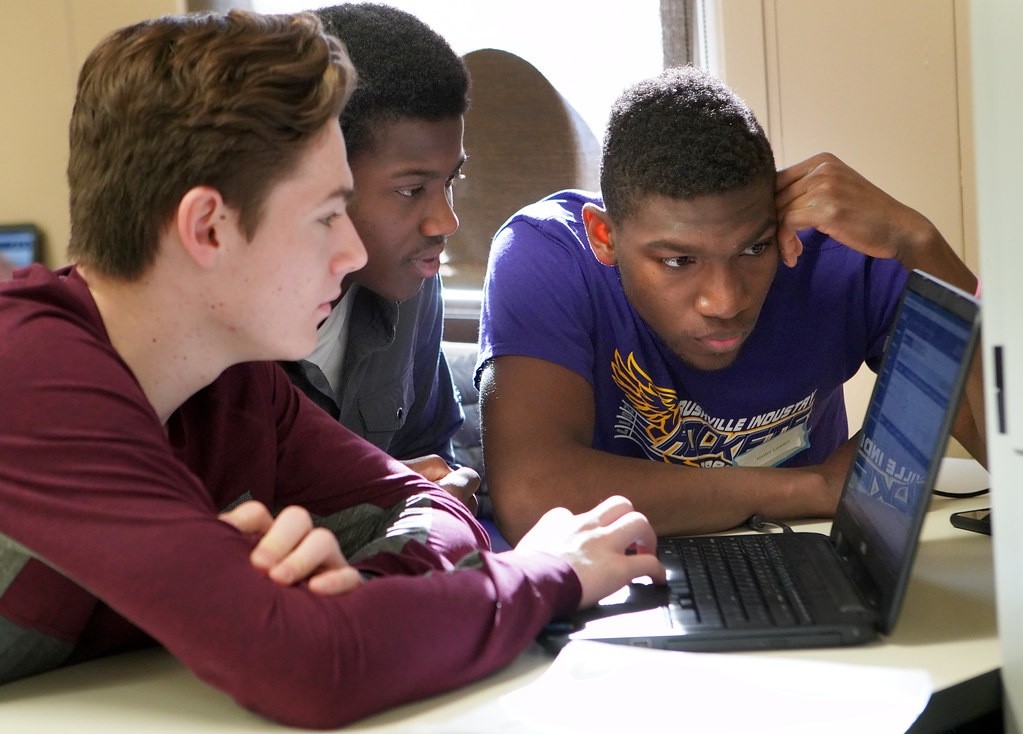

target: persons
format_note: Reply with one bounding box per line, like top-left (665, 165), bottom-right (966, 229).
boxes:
top-left (470, 63), bottom-right (990, 549)
top-left (0, 10), bottom-right (666, 733)
top-left (275, 1), bottom-right (483, 518)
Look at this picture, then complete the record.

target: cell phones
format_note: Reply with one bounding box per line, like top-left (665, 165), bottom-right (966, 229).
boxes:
top-left (950, 508), bottom-right (992, 536)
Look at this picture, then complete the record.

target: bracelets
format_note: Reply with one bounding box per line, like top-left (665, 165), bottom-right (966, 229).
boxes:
top-left (975, 278), bottom-right (982, 301)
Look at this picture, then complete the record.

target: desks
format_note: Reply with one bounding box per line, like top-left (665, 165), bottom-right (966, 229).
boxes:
top-left (0, 492), bottom-right (1023, 734)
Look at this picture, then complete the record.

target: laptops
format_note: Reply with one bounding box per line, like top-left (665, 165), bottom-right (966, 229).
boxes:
top-left (540, 267), bottom-right (982, 656)
top-left (0, 224), bottom-right (43, 268)
